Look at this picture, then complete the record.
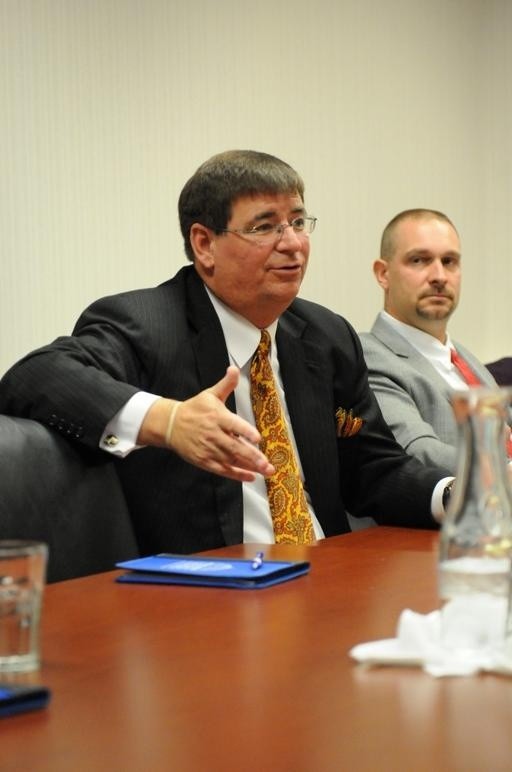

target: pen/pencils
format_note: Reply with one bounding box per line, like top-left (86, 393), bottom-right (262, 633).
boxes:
top-left (251, 552), bottom-right (263, 569)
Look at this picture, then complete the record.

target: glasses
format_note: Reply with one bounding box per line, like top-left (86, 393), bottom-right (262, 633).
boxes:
top-left (215, 215), bottom-right (318, 246)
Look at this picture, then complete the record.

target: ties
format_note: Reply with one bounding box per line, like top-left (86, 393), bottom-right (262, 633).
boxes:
top-left (250, 328), bottom-right (317, 545)
top-left (451, 348), bottom-right (512, 460)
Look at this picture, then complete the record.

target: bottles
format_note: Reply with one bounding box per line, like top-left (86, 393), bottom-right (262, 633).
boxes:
top-left (429, 383), bottom-right (512, 664)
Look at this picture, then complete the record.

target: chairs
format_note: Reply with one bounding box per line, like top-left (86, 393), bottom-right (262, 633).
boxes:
top-left (0, 410), bottom-right (140, 586)
top-left (485, 357), bottom-right (512, 407)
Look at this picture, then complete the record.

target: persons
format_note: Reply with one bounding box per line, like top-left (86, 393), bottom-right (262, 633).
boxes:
top-left (353, 207), bottom-right (512, 474)
top-left (3, 150), bottom-right (512, 558)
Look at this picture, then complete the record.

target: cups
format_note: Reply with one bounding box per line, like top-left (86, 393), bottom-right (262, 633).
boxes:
top-left (0, 535), bottom-right (49, 675)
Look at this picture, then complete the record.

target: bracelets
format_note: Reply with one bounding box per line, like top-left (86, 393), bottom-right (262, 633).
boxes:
top-left (440, 478), bottom-right (454, 515)
top-left (164, 397), bottom-right (181, 451)
